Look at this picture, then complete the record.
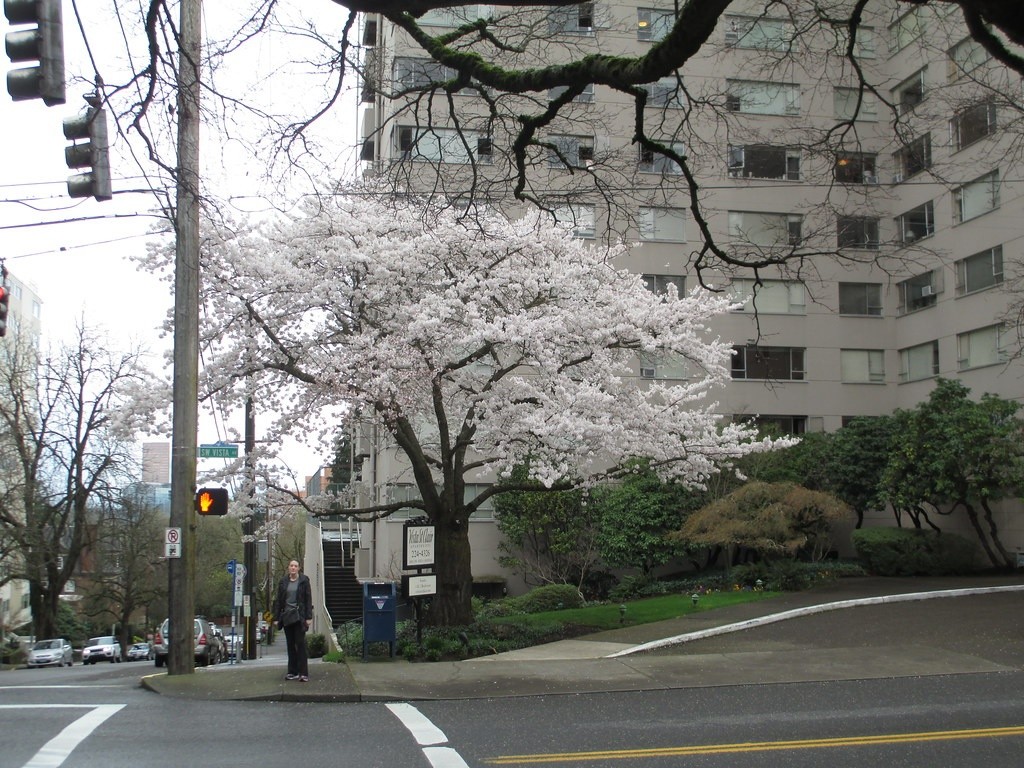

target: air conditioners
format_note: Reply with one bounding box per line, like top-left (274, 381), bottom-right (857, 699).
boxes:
top-left (892, 175), bottom-right (901, 184)
top-left (865, 176), bottom-right (879, 184)
top-left (921, 285), bottom-right (935, 297)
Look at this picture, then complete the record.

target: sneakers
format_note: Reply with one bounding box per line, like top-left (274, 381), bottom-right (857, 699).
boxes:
top-left (298, 675), bottom-right (308, 682)
top-left (285, 673), bottom-right (300, 680)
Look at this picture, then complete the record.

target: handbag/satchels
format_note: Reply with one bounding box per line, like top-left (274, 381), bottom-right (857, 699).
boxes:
top-left (281, 609), bottom-right (300, 626)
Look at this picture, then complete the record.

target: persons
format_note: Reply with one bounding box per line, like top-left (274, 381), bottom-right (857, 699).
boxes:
top-left (274, 558), bottom-right (312, 681)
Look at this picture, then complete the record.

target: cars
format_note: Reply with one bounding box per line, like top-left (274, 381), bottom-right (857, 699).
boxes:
top-left (154, 615), bottom-right (231, 668)
top-left (127, 643), bottom-right (155, 661)
top-left (82, 636), bottom-right (122, 665)
top-left (27, 639), bottom-right (73, 669)
top-left (256, 621), bottom-right (269, 643)
top-left (224, 636), bottom-right (243, 657)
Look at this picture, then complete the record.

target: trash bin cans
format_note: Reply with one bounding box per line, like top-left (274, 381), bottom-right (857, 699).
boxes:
top-left (363, 580), bottom-right (397, 660)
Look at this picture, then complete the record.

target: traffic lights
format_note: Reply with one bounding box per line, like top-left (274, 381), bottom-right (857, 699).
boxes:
top-left (3, 0), bottom-right (66, 107)
top-left (63, 107), bottom-right (112, 201)
top-left (0, 286), bottom-right (11, 337)
top-left (197, 488), bottom-right (228, 515)
